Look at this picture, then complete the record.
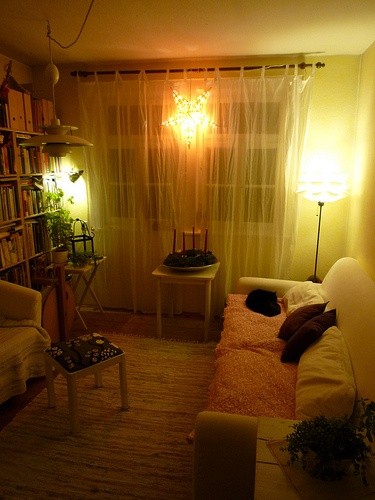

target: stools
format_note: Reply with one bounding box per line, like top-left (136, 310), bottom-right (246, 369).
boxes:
top-left (45, 332), bottom-right (129, 433)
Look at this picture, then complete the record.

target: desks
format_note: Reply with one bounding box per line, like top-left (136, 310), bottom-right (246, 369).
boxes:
top-left (254, 416), bottom-right (375, 500)
top-left (41, 256), bottom-right (107, 330)
top-left (152, 261), bottom-right (221, 341)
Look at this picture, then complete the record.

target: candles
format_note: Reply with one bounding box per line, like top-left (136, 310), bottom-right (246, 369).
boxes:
top-left (192, 226), bottom-right (195, 248)
top-left (205, 228), bottom-right (207, 251)
top-left (183, 232), bottom-right (185, 257)
top-left (172, 229), bottom-right (176, 252)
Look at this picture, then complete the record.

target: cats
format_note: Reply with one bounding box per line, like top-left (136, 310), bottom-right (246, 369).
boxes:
top-left (245, 288), bottom-right (281, 317)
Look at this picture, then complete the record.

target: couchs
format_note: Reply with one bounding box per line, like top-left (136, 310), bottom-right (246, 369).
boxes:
top-left (186, 257), bottom-right (375, 500)
top-left (0, 280), bottom-right (53, 406)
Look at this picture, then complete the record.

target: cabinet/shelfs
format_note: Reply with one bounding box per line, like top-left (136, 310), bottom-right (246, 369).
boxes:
top-left (0, 88), bottom-right (62, 288)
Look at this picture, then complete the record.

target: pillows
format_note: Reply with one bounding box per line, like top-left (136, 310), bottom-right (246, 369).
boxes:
top-left (277, 301), bottom-right (330, 339)
top-left (282, 280), bottom-right (329, 316)
top-left (280, 309), bottom-right (337, 363)
top-left (295, 326), bottom-right (357, 427)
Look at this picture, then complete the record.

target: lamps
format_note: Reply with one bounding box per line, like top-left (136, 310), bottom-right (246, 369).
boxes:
top-left (19, 24), bottom-right (94, 158)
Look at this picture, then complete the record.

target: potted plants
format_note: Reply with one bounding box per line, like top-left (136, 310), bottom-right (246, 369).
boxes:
top-left (30, 167), bottom-right (84, 263)
top-left (281, 397), bottom-right (375, 489)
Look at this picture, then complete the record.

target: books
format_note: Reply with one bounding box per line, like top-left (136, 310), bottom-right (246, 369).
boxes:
top-left (0, 139), bottom-right (44, 175)
top-left (0, 178), bottom-right (48, 221)
top-left (0, 218), bottom-right (54, 288)
